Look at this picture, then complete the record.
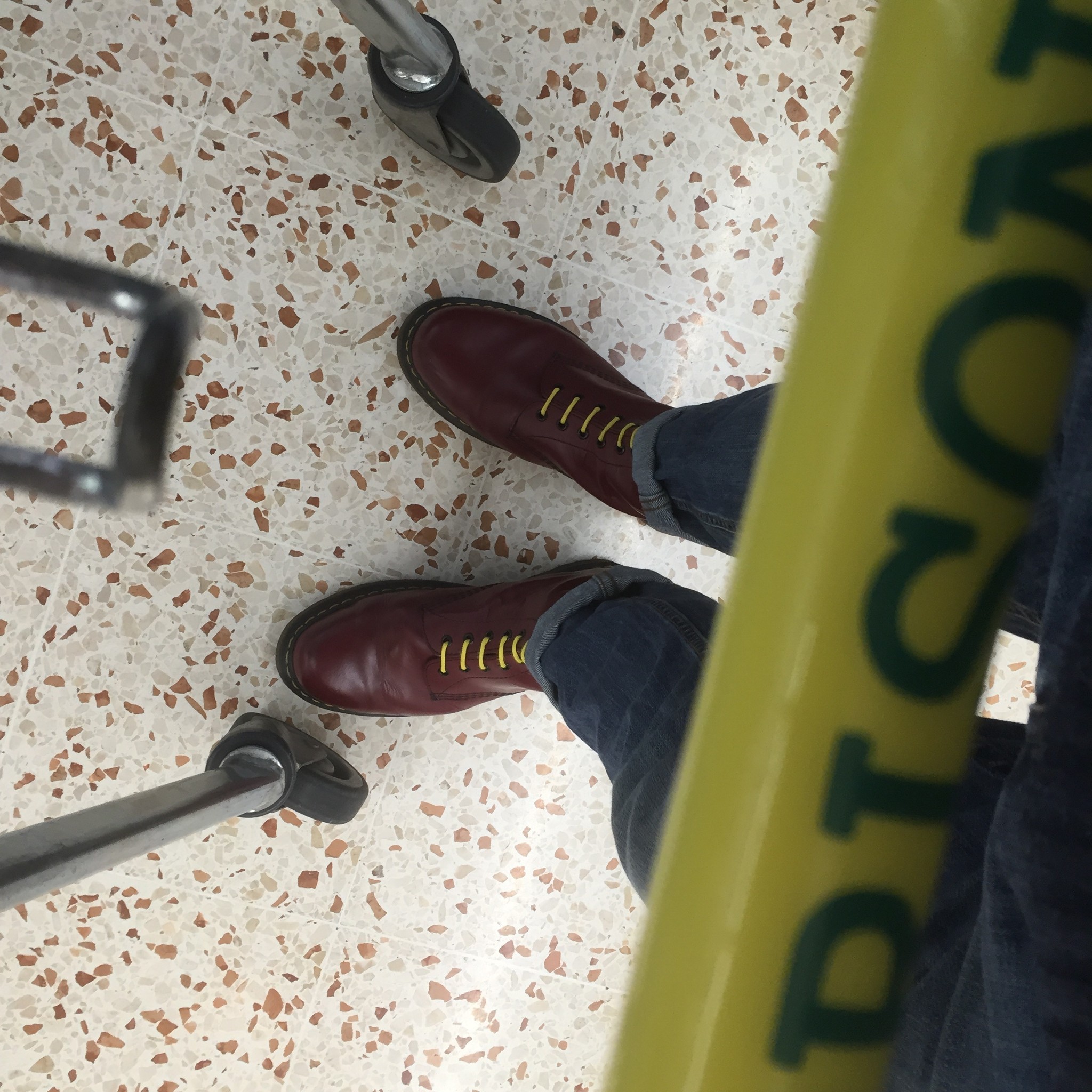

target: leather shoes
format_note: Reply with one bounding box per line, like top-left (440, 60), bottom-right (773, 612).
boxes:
top-left (275, 557), bottom-right (624, 719)
top-left (397, 300), bottom-right (675, 522)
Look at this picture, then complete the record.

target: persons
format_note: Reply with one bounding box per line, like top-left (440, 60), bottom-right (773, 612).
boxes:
top-left (274, 298), bottom-right (776, 909)
